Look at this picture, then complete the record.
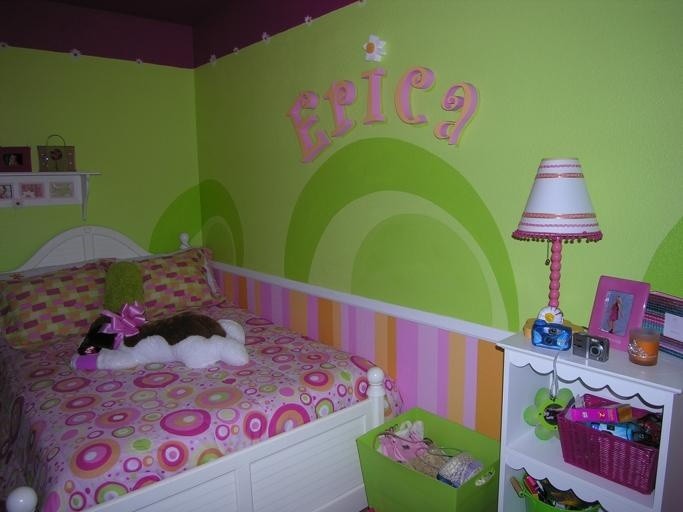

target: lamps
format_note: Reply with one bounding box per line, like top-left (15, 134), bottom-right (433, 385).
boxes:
top-left (512, 157), bottom-right (602, 338)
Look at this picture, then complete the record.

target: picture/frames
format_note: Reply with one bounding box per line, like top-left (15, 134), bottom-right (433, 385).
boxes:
top-left (0, 147), bottom-right (32, 172)
top-left (587, 275), bottom-right (650, 352)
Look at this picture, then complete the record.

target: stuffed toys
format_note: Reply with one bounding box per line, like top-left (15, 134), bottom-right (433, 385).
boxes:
top-left (71, 313), bottom-right (250, 373)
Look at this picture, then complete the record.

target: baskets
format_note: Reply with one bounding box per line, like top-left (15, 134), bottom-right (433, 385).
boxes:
top-left (556, 393), bottom-right (658, 497)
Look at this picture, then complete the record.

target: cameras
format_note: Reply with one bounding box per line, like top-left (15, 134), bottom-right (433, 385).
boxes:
top-left (573, 332), bottom-right (609, 362)
top-left (532, 319), bottom-right (572, 350)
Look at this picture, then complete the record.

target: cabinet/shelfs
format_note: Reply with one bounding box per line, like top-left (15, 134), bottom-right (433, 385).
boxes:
top-left (497, 326), bottom-right (683, 512)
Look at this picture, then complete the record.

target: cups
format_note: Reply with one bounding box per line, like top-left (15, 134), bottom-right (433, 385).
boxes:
top-left (628, 326), bottom-right (660, 365)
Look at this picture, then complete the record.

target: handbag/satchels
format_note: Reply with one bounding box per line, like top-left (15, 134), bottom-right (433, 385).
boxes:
top-left (36, 134), bottom-right (76, 173)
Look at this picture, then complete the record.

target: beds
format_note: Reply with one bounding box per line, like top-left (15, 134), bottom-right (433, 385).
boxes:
top-left (0, 225), bottom-right (402, 512)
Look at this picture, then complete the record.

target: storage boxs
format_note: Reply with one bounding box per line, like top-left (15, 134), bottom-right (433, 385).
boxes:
top-left (509, 472), bottom-right (600, 512)
top-left (356, 406), bottom-right (501, 512)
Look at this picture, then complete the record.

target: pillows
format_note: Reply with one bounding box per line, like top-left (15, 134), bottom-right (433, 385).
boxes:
top-left (130, 247), bottom-right (226, 326)
top-left (0, 258), bottom-right (116, 351)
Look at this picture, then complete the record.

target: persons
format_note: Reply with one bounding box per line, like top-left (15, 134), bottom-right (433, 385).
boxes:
top-left (603, 295), bottom-right (624, 334)
top-left (7, 154), bottom-right (18, 166)
